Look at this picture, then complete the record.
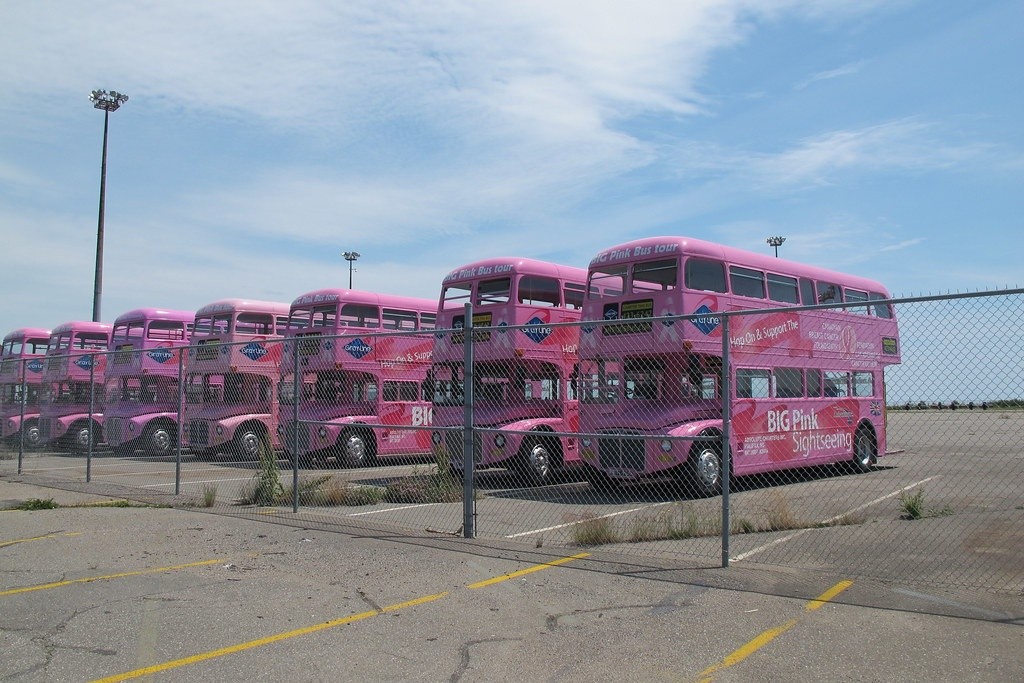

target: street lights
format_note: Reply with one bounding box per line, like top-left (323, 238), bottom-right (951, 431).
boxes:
top-left (341, 251), bottom-right (361, 289)
top-left (766, 236), bottom-right (786, 257)
top-left (88, 89), bottom-right (129, 322)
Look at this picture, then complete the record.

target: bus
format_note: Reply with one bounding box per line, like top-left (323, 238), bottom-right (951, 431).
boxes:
top-left (276, 288), bottom-right (541, 470)
top-left (420, 257), bottom-right (720, 488)
top-left (103, 307), bottom-right (311, 457)
top-left (181, 298), bottom-right (375, 462)
top-left (567, 235), bottom-right (901, 499)
top-left (1, 328), bottom-right (177, 451)
top-left (38, 321), bottom-right (224, 454)
